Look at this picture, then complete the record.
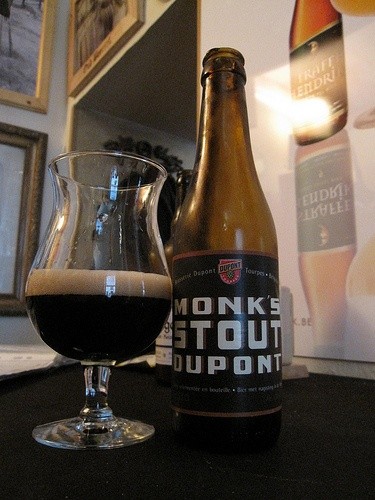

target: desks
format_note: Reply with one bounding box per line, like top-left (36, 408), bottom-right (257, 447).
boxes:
top-left (0, 364), bottom-right (375, 500)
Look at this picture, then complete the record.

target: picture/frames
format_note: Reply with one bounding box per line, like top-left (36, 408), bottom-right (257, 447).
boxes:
top-left (67, 0), bottom-right (145, 96)
top-left (0, 121), bottom-right (51, 316)
top-left (0, 0), bottom-right (57, 115)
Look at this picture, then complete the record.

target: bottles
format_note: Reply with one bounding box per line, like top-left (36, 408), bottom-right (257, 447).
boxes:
top-left (292, 129), bottom-right (358, 359)
top-left (155, 169), bottom-right (193, 388)
top-left (288, 0), bottom-right (348, 145)
top-left (171, 48), bottom-right (282, 452)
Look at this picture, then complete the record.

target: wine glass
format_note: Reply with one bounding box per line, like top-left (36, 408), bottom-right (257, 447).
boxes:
top-left (24, 149), bottom-right (172, 450)
